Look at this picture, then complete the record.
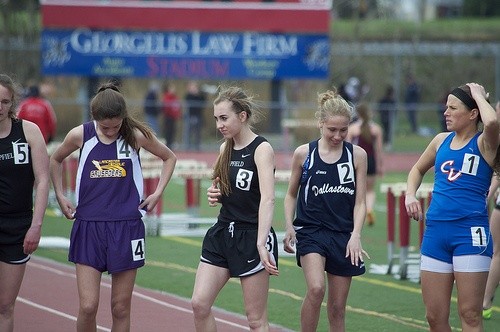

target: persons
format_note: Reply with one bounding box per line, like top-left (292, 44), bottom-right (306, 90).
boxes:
top-left (145, 82), bottom-right (208, 152)
top-left (338, 75), bottom-right (500, 332)
top-left (0, 73), bottom-right (51, 332)
top-left (190, 85), bottom-right (280, 332)
top-left (12, 81), bottom-right (56, 145)
top-left (284, 90), bottom-right (369, 332)
top-left (49, 82), bottom-right (178, 332)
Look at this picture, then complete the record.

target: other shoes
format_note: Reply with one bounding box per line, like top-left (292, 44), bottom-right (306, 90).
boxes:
top-left (482, 307), bottom-right (492, 319)
top-left (366, 210), bottom-right (374, 227)
